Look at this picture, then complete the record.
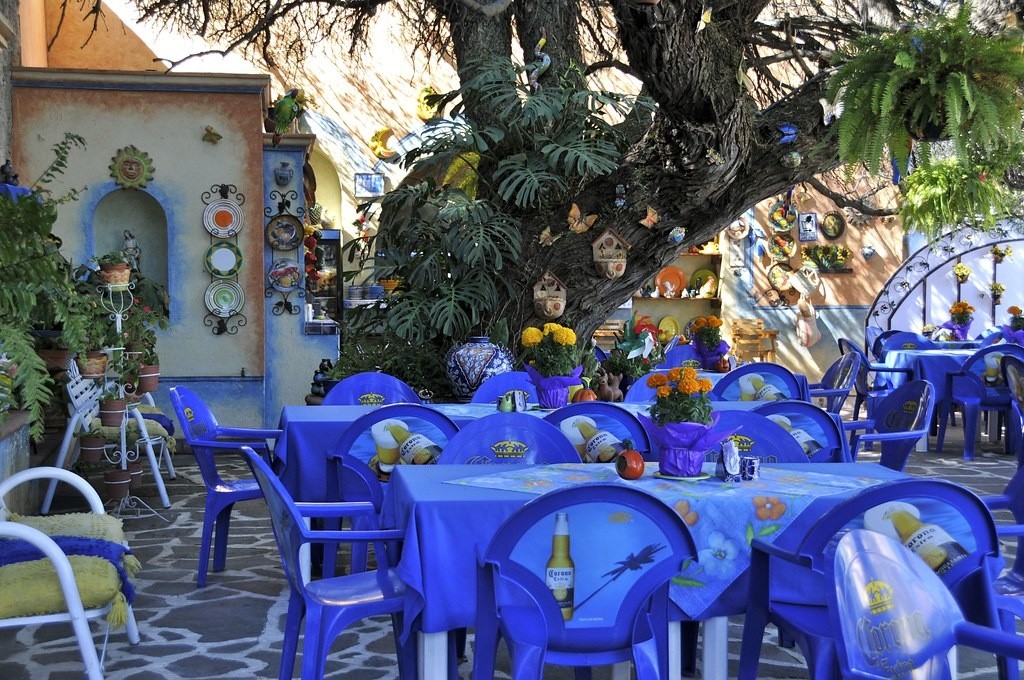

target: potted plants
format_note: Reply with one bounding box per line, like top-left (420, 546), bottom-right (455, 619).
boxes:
top-left (73, 253), bottom-right (160, 496)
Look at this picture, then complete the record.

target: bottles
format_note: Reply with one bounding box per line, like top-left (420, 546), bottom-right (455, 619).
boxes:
top-left (386, 421), bottom-right (444, 465)
top-left (748, 375), bottom-right (788, 401)
top-left (574, 417), bottom-right (626, 464)
top-left (771, 417), bottom-right (824, 459)
top-left (446, 336), bottom-right (517, 403)
top-left (544, 512), bottom-right (575, 621)
top-left (887, 505), bottom-right (969, 578)
top-left (992, 353), bottom-right (1024, 410)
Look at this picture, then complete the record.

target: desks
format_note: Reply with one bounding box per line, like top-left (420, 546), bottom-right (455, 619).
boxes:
top-left (379, 464), bottom-right (1004, 680)
top-left (277, 398), bottom-right (852, 586)
top-left (934, 341), bottom-right (982, 349)
top-left (653, 368), bottom-right (811, 405)
top-left (886, 348), bottom-right (1000, 443)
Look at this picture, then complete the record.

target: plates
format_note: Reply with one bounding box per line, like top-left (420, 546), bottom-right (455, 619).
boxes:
top-left (726, 202), bottom-right (845, 292)
top-left (203, 199), bottom-right (246, 319)
top-left (654, 266), bottom-right (719, 341)
top-left (266, 214), bottom-right (304, 292)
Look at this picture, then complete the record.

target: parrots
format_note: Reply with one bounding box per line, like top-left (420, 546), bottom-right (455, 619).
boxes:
top-left (271, 89), bottom-right (300, 149)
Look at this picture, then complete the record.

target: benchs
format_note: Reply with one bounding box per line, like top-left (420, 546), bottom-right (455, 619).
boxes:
top-left (40, 372), bottom-right (176, 510)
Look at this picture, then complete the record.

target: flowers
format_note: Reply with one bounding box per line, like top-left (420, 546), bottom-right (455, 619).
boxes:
top-left (647, 365), bottom-right (713, 427)
top-left (799, 241), bottom-right (852, 270)
top-left (690, 314), bottom-right (722, 351)
top-left (949, 246), bottom-right (1024, 330)
top-left (521, 323), bottom-right (579, 380)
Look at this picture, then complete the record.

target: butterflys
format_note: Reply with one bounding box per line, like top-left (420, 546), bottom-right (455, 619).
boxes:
top-left (538, 200), bottom-right (663, 247)
top-left (779, 86), bottom-right (847, 144)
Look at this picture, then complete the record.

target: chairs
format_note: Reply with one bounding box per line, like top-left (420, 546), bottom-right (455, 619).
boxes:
top-left (0, 467), bottom-right (143, 680)
top-left (165, 318), bottom-right (1024, 680)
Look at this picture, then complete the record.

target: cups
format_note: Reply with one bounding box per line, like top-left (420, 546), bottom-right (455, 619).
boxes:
top-left (739, 373), bottom-right (764, 401)
top-left (862, 500), bottom-right (920, 543)
top-left (741, 456), bottom-right (761, 481)
top-left (559, 415), bottom-right (596, 461)
top-left (984, 351), bottom-right (1005, 383)
top-left (348, 279), bottom-right (400, 300)
top-left (497, 395), bottom-right (504, 410)
top-left (371, 419), bottom-right (410, 474)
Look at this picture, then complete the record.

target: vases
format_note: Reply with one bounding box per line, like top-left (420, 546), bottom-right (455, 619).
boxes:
top-left (937, 318), bottom-right (974, 341)
top-left (690, 336), bottom-right (730, 369)
top-left (349, 278), bottom-right (398, 300)
top-left (1003, 325), bottom-right (1024, 347)
top-left (636, 411), bottom-right (744, 477)
top-left (521, 359), bottom-right (583, 411)
